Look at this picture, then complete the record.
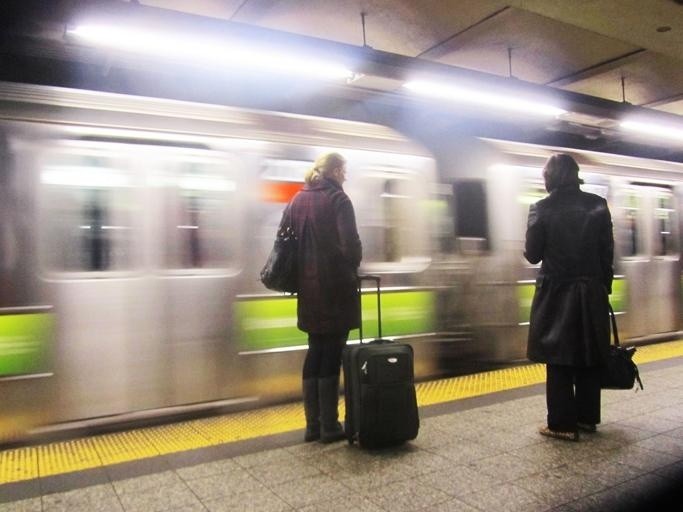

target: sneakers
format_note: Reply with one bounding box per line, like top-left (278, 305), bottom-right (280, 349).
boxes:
top-left (579, 420), bottom-right (598, 434)
top-left (540, 422), bottom-right (582, 442)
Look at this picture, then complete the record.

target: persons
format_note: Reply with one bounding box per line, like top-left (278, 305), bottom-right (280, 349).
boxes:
top-left (278, 151), bottom-right (363, 443)
top-left (522, 153), bottom-right (615, 441)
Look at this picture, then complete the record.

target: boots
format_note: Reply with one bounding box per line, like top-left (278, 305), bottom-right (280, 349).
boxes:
top-left (318, 375), bottom-right (345, 445)
top-left (300, 375), bottom-right (318, 442)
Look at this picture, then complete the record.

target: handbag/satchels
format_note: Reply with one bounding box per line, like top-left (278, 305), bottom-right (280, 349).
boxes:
top-left (258, 232), bottom-right (298, 295)
top-left (600, 341), bottom-right (642, 390)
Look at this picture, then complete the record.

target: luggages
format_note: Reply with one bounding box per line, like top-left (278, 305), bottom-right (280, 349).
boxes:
top-left (341, 273), bottom-right (420, 453)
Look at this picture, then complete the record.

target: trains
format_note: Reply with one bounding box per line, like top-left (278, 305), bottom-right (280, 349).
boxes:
top-left (0, 76), bottom-right (681, 448)
